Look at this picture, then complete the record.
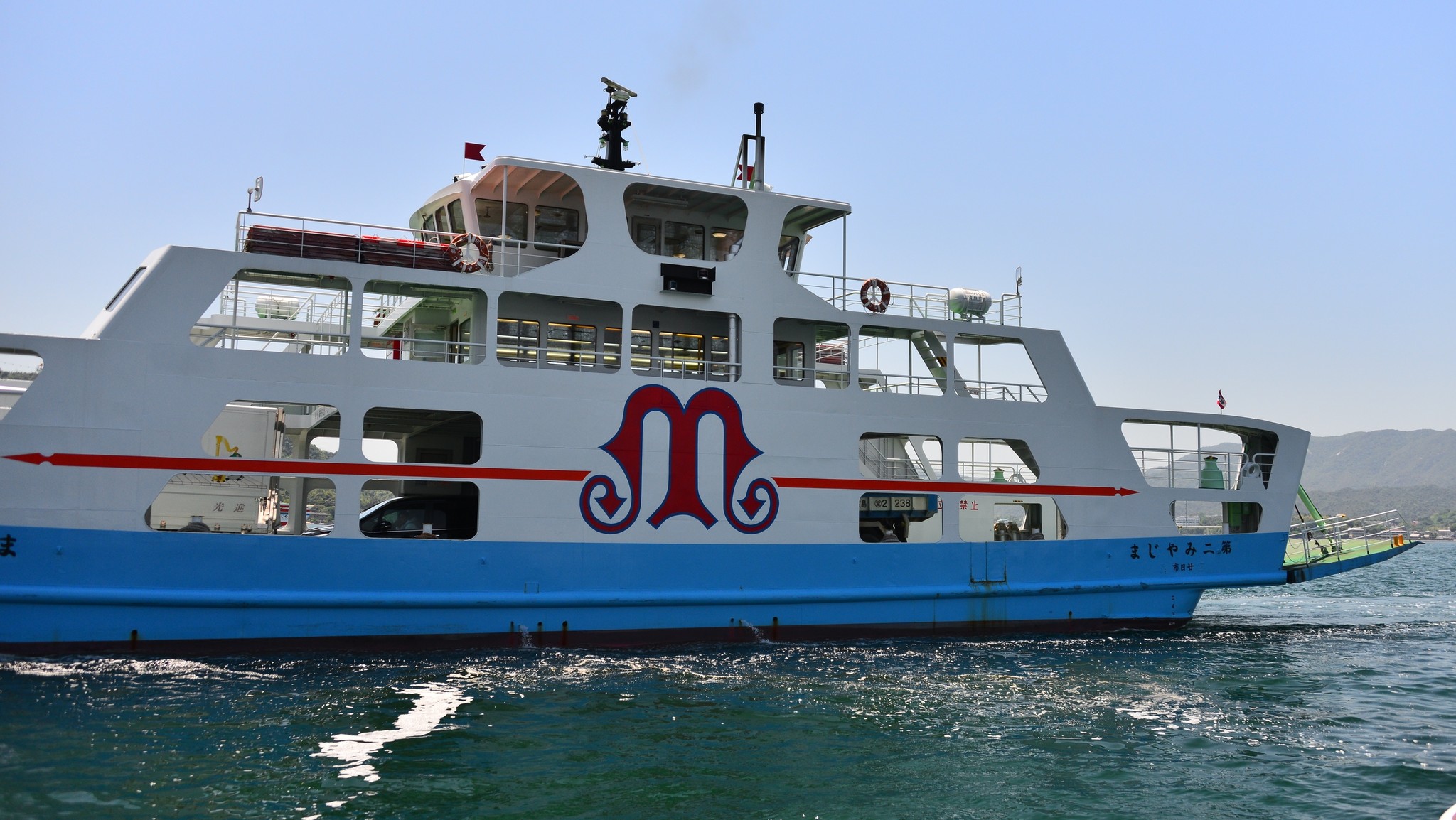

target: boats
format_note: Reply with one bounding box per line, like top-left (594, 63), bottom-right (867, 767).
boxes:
top-left (0, 74), bottom-right (1425, 660)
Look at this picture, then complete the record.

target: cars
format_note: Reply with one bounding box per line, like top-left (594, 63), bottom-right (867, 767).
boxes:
top-left (301, 494), bottom-right (479, 541)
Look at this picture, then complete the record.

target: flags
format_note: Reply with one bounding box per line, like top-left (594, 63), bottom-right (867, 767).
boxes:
top-left (1216, 390), bottom-right (1226, 409)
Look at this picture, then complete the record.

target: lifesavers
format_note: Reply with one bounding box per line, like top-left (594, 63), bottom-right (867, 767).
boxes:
top-left (449, 232), bottom-right (489, 273)
top-left (860, 278), bottom-right (891, 313)
